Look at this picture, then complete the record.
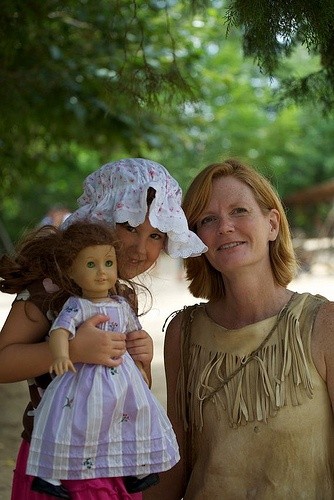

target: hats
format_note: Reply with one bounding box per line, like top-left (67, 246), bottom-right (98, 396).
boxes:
top-left (60, 158), bottom-right (209, 259)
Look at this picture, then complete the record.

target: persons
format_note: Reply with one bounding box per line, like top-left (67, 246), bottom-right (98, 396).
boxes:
top-left (139, 159), bottom-right (334, 500)
top-left (0, 157), bottom-right (208, 500)
top-left (0, 221), bottom-right (182, 500)
